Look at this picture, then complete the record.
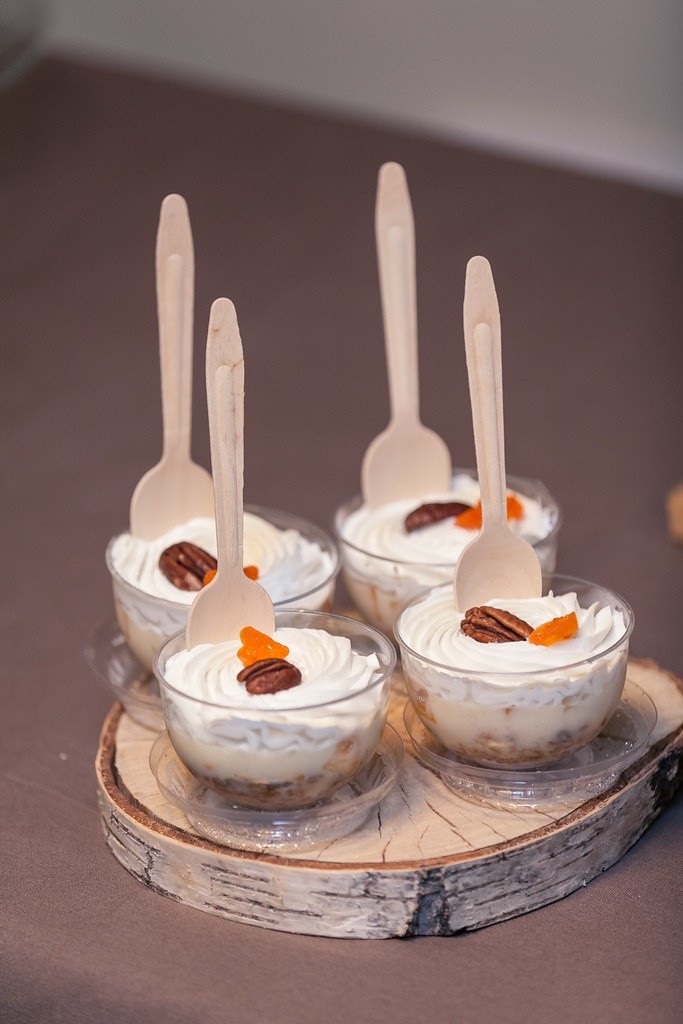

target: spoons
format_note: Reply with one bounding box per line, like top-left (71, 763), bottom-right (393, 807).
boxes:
top-left (127, 194), bottom-right (218, 540)
top-left (452, 256), bottom-right (543, 614)
top-left (358, 162), bottom-right (452, 510)
top-left (181, 297), bottom-right (275, 649)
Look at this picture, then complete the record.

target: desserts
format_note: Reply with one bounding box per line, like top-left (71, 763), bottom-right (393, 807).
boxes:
top-left (161, 626), bottom-right (388, 813)
top-left (343, 472), bottom-right (556, 649)
top-left (397, 583), bottom-right (629, 769)
top-left (111, 509), bottom-right (339, 677)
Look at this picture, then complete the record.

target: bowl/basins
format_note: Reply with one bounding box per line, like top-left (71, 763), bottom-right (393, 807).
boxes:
top-left (392, 571), bottom-right (636, 772)
top-left (151, 608), bottom-right (399, 809)
top-left (101, 503), bottom-right (343, 675)
top-left (330, 463), bottom-right (563, 646)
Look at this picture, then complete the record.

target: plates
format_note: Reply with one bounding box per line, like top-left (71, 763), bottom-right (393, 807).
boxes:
top-left (397, 674), bottom-right (662, 812)
top-left (145, 716), bottom-right (409, 854)
top-left (82, 610), bottom-right (168, 731)
